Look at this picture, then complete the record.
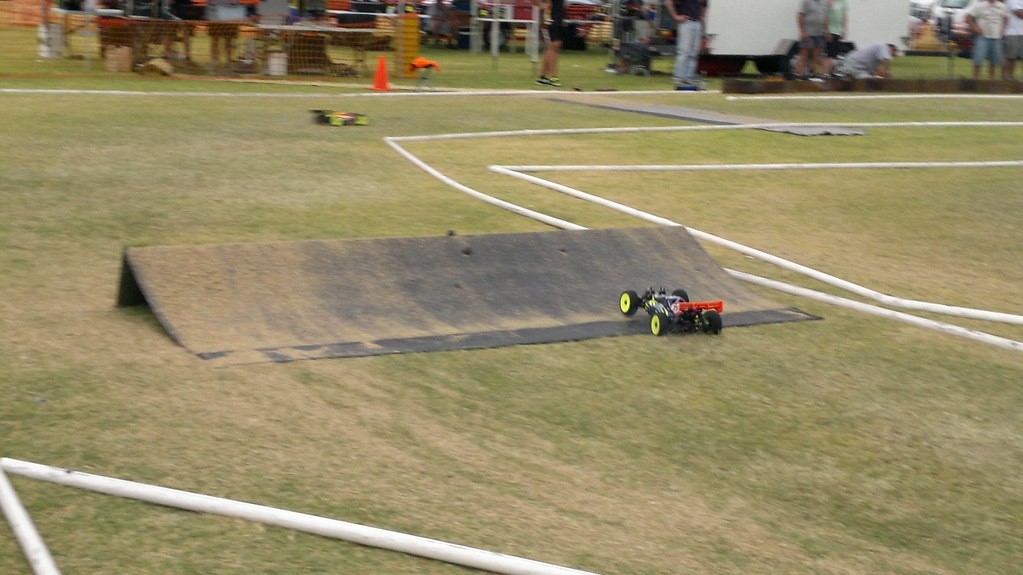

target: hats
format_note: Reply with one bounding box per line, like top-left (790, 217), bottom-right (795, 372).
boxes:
top-left (887, 41), bottom-right (898, 51)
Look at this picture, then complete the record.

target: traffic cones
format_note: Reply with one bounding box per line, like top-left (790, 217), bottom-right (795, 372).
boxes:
top-left (366, 55), bottom-right (396, 92)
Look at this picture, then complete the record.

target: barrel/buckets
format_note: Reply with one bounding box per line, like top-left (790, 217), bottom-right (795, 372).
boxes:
top-left (267, 53), bottom-right (288, 76)
top-left (37, 22), bottom-right (62, 58)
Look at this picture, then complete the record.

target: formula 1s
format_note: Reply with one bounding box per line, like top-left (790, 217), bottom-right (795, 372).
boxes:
top-left (620, 287), bottom-right (724, 336)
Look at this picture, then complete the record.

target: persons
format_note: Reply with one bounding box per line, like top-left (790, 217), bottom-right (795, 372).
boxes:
top-left (35, 0), bottom-right (290, 75)
top-left (425, 0), bottom-right (457, 50)
top-left (965, 0), bottom-right (1023, 80)
top-left (831, 42), bottom-right (897, 80)
top-left (796, 0), bottom-right (847, 81)
top-left (664, 0), bottom-right (709, 90)
top-left (533, 0), bottom-right (565, 87)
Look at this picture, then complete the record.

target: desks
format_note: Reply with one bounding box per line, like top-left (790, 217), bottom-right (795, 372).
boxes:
top-left (564, 20), bottom-right (605, 67)
top-left (474, 18), bottom-right (537, 53)
top-left (256, 24), bottom-right (377, 79)
top-left (327, 10), bottom-right (434, 46)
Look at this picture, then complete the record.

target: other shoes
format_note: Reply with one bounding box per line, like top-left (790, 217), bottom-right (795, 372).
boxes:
top-left (794, 74), bottom-right (825, 83)
top-left (534, 76), bottom-right (561, 87)
top-left (676, 83), bottom-right (706, 90)
